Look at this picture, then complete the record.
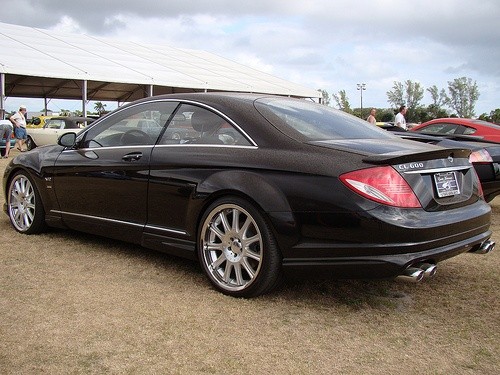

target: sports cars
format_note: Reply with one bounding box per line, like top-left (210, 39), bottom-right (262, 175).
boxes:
top-left (377, 124), bottom-right (500, 205)
top-left (410, 116), bottom-right (500, 143)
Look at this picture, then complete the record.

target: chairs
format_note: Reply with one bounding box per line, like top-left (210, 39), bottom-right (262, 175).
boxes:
top-left (190, 110), bottom-right (226, 145)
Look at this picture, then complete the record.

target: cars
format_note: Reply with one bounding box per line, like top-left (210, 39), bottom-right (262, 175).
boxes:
top-left (25, 116), bottom-right (96, 151)
top-left (0, 131), bottom-right (17, 153)
top-left (1, 90), bottom-right (497, 299)
top-left (26, 115), bottom-right (50, 128)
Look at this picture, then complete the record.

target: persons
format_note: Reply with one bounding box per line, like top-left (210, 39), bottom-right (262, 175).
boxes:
top-left (367, 109), bottom-right (376, 124)
top-left (394, 106), bottom-right (406, 129)
top-left (0, 120), bottom-right (13, 159)
top-left (10, 106), bottom-right (28, 152)
